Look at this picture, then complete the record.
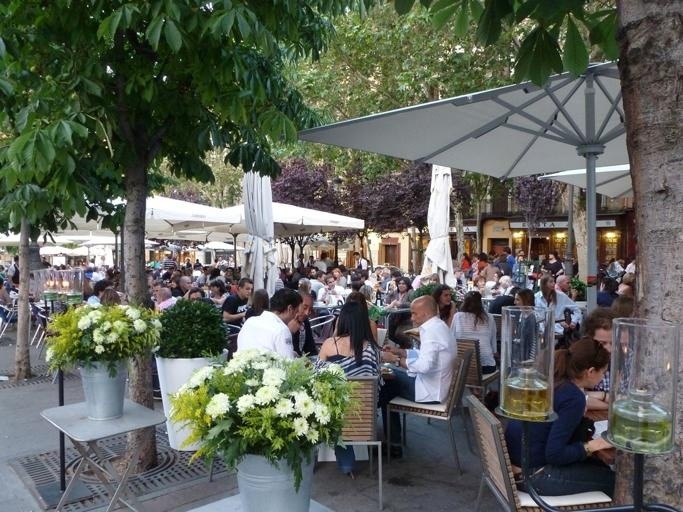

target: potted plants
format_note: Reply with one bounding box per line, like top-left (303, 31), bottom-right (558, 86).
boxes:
top-left (154, 297), bottom-right (230, 452)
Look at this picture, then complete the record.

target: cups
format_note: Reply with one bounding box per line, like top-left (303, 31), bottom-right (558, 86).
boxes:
top-left (501, 304), bottom-right (555, 417)
top-left (607, 316), bottom-right (679, 452)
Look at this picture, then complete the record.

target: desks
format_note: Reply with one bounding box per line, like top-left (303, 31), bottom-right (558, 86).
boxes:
top-left (185, 492), bottom-right (335, 512)
top-left (40, 398), bottom-right (168, 512)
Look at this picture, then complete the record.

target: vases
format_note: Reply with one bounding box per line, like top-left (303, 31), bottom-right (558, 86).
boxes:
top-left (79, 357), bottom-right (131, 423)
top-left (233, 449), bottom-right (319, 512)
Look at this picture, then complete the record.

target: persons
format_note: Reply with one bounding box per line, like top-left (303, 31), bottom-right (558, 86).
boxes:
top-left (144, 251), bottom-right (639, 502)
top-left (0, 254), bottom-right (122, 331)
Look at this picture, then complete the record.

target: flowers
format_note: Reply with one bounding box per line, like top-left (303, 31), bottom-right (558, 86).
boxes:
top-left (166, 346), bottom-right (366, 495)
top-left (42, 298), bottom-right (166, 379)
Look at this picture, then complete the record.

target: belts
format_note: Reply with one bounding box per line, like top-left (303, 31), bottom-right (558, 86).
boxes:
top-left (514, 466), bottom-right (543, 481)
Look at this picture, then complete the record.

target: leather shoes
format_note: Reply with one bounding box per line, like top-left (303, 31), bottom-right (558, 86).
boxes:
top-left (373, 445), bottom-right (402, 458)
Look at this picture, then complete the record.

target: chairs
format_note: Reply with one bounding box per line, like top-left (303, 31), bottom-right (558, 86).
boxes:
top-left (0, 299), bottom-right (63, 349)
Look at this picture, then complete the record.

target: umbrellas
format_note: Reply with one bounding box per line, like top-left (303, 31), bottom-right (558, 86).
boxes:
top-left (296, 59), bottom-right (630, 315)
top-left (536, 163), bottom-right (636, 201)
